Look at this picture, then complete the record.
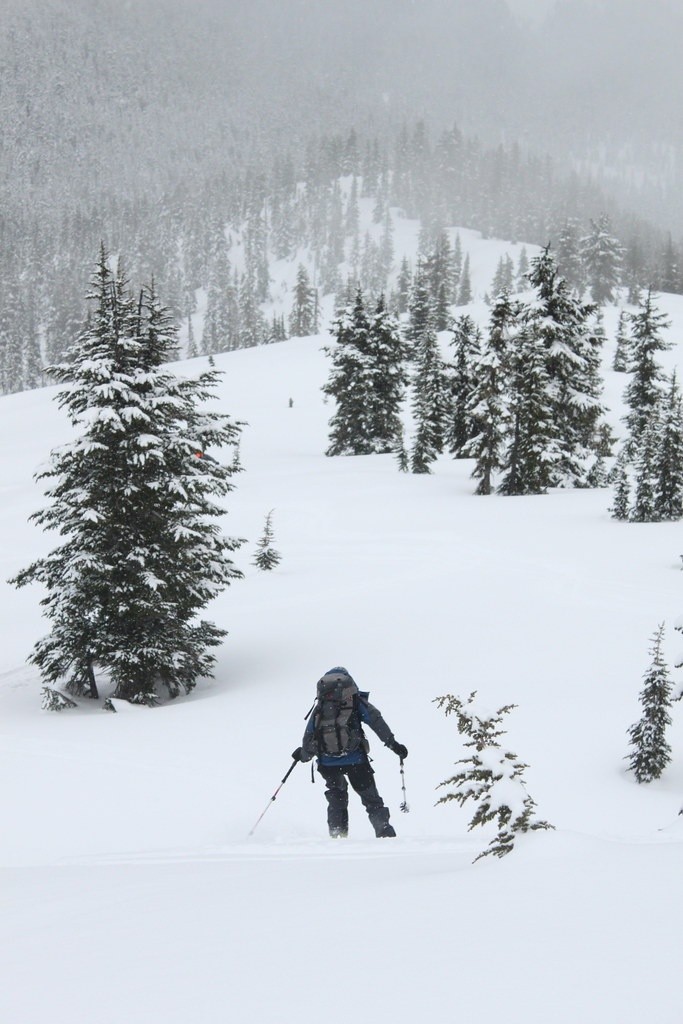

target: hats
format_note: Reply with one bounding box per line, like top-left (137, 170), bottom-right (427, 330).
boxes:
top-left (324, 665), bottom-right (350, 676)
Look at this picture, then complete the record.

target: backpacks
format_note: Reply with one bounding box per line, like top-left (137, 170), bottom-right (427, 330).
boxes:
top-left (304, 672), bottom-right (360, 757)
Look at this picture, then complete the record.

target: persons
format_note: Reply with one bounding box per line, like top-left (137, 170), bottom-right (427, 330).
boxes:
top-left (292, 667), bottom-right (408, 839)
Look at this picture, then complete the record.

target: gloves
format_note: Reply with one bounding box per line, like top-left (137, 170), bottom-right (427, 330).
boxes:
top-left (292, 747), bottom-right (313, 762)
top-left (388, 741), bottom-right (408, 759)
